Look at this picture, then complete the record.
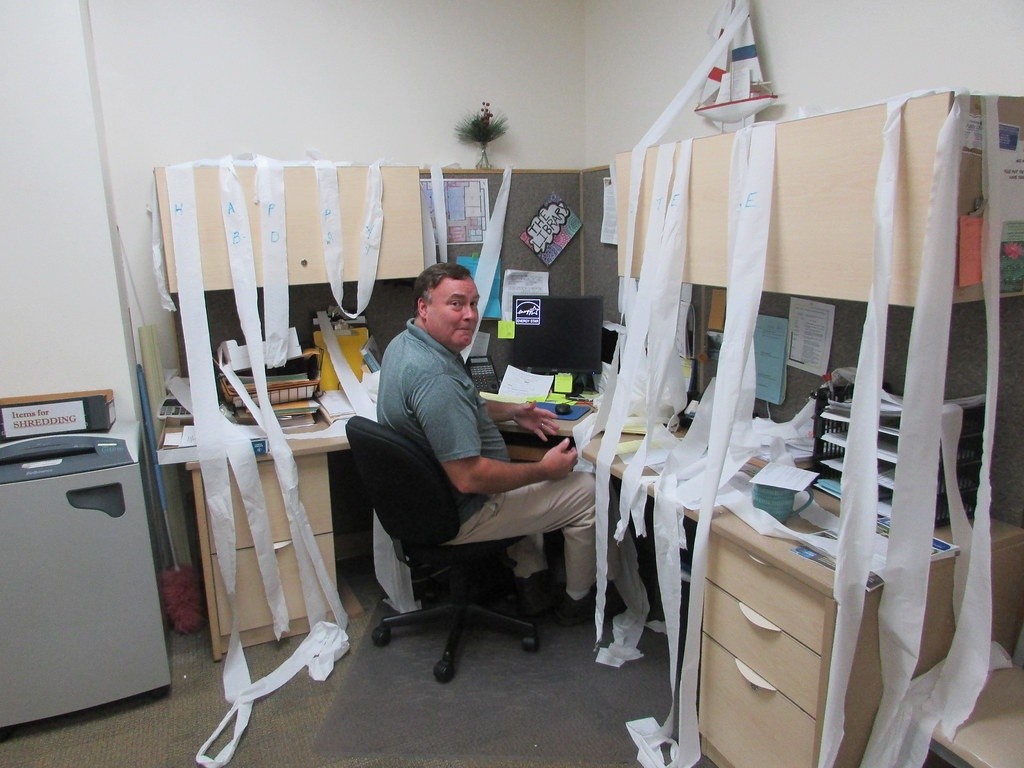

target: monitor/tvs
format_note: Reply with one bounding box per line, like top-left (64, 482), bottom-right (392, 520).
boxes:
top-left (513, 295), bottom-right (603, 398)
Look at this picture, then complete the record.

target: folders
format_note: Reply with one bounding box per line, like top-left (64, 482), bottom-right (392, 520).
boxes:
top-left (359, 335), bottom-right (384, 373)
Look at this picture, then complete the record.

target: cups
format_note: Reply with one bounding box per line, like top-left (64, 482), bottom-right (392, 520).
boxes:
top-left (751, 481), bottom-right (815, 524)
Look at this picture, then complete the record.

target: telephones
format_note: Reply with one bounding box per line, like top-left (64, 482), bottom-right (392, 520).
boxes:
top-left (464, 355), bottom-right (500, 394)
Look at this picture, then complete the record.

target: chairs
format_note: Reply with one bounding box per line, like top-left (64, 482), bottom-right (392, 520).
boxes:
top-left (342, 415), bottom-right (539, 682)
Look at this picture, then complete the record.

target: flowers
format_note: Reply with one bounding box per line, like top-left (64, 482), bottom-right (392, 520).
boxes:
top-left (455, 98), bottom-right (510, 147)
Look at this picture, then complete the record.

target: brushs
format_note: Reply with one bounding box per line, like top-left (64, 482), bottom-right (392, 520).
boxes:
top-left (136, 364), bottom-right (205, 636)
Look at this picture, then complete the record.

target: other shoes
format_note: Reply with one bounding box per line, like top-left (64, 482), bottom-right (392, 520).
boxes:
top-left (519, 591), bottom-right (546, 616)
top-left (553, 583), bottom-right (624, 625)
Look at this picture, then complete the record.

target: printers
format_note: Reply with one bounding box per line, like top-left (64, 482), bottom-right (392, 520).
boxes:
top-left (0, 434), bottom-right (171, 729)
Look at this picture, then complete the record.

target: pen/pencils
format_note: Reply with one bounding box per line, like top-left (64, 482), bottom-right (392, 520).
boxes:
top-left (576, 401), bottom-right (593, 404)
top-left (569, 396), bottom-right (594, 402)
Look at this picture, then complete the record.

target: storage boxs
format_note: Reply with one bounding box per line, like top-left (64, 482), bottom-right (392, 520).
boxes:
top-left (0, 389), bottom-right (117, 445)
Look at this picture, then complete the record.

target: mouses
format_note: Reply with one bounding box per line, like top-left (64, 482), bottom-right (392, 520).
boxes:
top-left (555, 403), bottom-right (570, 415)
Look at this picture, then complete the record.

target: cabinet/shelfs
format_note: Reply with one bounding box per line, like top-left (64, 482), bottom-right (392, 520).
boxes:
top-left (808, 387), bottom-right (985, 529)
top-left (614, 91), bottom-right (1024, 306)
top-left (155, 163), bottom-right (425, 293)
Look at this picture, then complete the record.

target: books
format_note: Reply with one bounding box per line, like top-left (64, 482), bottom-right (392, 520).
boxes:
top-left (359, 336), bottom-right (381, 372)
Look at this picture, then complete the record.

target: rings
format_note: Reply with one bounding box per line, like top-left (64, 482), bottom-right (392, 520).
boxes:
top-left (539, 422), bottom-right (543, 429)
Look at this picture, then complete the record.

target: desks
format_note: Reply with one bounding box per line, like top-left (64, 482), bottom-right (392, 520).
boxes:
top-left (156, 394), bottom-right (1024, 768)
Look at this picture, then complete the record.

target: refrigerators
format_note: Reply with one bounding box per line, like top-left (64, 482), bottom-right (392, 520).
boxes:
top-left (1, 0), bottom-right (173, 733)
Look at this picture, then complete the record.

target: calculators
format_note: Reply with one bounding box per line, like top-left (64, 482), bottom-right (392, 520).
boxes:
top-left (157, 395), bottom-right (194, 424)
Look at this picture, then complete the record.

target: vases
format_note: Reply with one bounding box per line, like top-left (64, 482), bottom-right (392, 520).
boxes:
top-left (472, 147), bottom-right (493, 170)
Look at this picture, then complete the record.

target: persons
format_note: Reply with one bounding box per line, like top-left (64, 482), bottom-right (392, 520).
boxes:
top-left (378, 261), bottom-right (622, 625)
